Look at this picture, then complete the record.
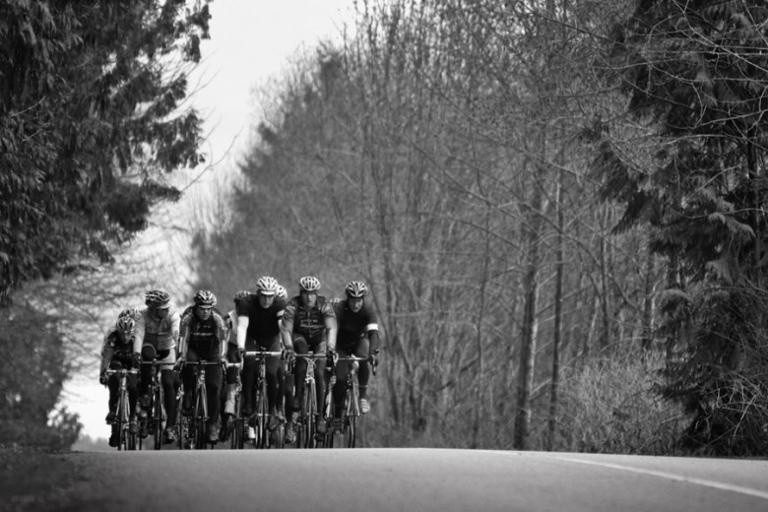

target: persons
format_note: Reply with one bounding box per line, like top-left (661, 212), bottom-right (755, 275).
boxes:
top-left (101, 276), bottom-right (338, 448)
top-left (333, 280), bottom-right (379, 432)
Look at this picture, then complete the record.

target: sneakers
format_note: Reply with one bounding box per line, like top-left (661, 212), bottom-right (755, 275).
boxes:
top-left (359, 398), bottom-right (370, 414)
top-left (106, 412), bottom-right (343, 447)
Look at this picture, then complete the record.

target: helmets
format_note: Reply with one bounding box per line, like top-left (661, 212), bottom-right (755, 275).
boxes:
top-left (115, 277), bottom-right (368, 334)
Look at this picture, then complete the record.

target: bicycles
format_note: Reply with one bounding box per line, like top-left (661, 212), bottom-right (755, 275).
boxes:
top-left (104, 348), bottom-right (377, 450)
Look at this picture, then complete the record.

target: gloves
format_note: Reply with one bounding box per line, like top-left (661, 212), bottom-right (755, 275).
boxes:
top-left (100, 352), bottom-right (377, 385)
